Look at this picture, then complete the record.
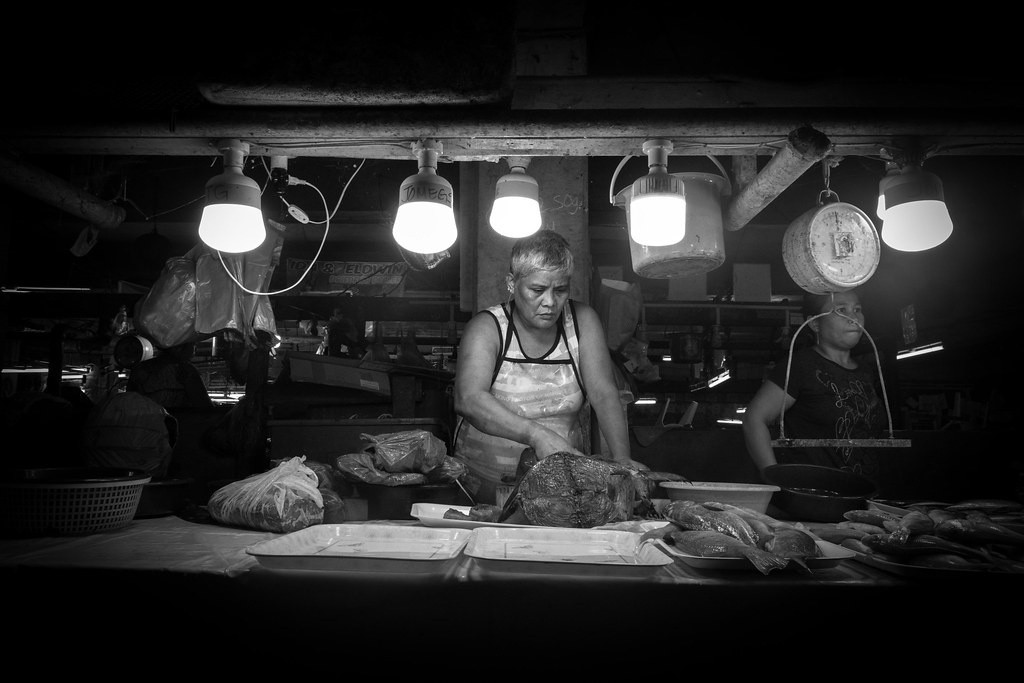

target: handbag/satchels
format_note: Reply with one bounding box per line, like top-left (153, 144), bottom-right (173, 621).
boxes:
top-left (133, 213), bottom-right (288, 348)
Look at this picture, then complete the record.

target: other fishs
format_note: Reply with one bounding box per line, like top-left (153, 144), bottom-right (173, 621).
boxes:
top-left (633, 501), bottom-right (1024, 575)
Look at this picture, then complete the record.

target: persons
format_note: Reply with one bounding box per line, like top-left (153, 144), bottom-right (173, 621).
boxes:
top-left (742, 289), bottom-right (890, 500)
top-left (453, 230), bottom-right (651, 508)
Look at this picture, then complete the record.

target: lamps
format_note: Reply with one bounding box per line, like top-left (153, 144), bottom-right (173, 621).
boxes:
top-left (489, 157), bottom-right (543, 238)
top-left (196, 140), bottom-right (266, 254)
top-left (881, 157), bottom-right (954, 252)
top-left (877, 162), bottom-right (887, 220)
top-left (391, 141), bottom-right (458, 254)
top-left (628, 141), bottom-right (686, 246)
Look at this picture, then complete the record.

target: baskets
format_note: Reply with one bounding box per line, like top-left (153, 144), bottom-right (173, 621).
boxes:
top-left (0, 477), bottom-right (152, 536)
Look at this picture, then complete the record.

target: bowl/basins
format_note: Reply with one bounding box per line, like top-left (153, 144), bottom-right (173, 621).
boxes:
top-left (762, 463), bottom-right (880, 523)
top-left (657, 482), bottom-right (781, 516)
top-left (0, 465), bottom-right (148, 483)
